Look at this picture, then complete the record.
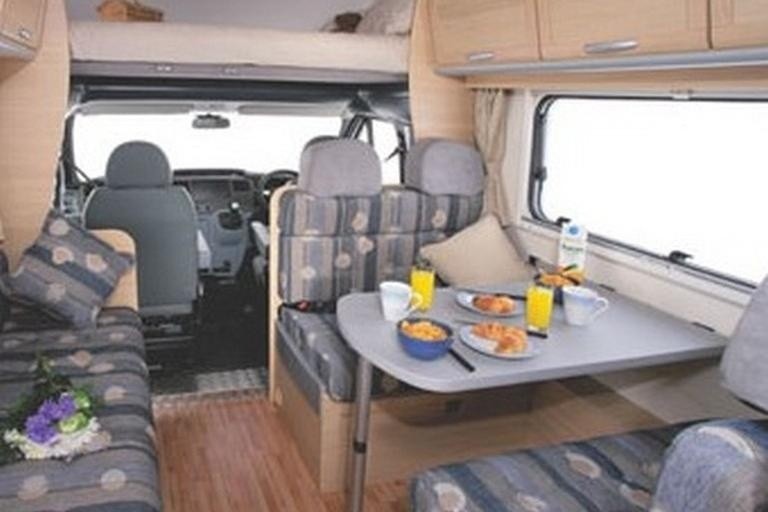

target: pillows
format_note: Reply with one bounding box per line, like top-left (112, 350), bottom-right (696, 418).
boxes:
top-left (419, 212), bottom-right (539, 286)
top-left (0, 208), bottom-right (133, 334)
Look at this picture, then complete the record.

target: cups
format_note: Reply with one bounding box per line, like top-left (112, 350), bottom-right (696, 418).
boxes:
top-left (526, 281), bottom-right (554, 332)
top-left (562, 285), bottom-right (609, 325)
top-left (411, 266), bottom-right (436, 313)
top-left (379, 282), bottom-right (424, 322)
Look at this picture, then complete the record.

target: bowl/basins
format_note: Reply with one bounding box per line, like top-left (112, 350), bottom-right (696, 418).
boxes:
top-left (533, 272), bottom-right (580, 306)
top-left (397, 317), bottom-right (454, 359)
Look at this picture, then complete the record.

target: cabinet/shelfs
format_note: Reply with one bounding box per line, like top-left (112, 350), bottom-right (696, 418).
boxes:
top-left (428, 0), bottom-right (768, 77)
top-left (0, 0), bottom-right (47, 60)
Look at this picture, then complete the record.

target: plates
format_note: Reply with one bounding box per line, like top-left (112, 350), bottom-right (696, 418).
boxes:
top-left (459, 323), bottom-right (543, 360)
top-left (455, 290), bottom-right (525, 317)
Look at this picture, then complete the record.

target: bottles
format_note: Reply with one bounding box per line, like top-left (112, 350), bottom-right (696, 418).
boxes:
top-left (558, 214), bottom-right (587, 270)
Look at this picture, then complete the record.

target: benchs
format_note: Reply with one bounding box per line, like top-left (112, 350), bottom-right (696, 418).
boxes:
top-left (411, 277), bottom-right (768, 512)
top-left (275, 136), bottom-right (527, 402)
top-left (0, 248), bottom-right (163, 512)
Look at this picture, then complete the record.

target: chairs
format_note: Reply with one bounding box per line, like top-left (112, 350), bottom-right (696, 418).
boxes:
top-left (81, 142), bottom-right (212, 320)
